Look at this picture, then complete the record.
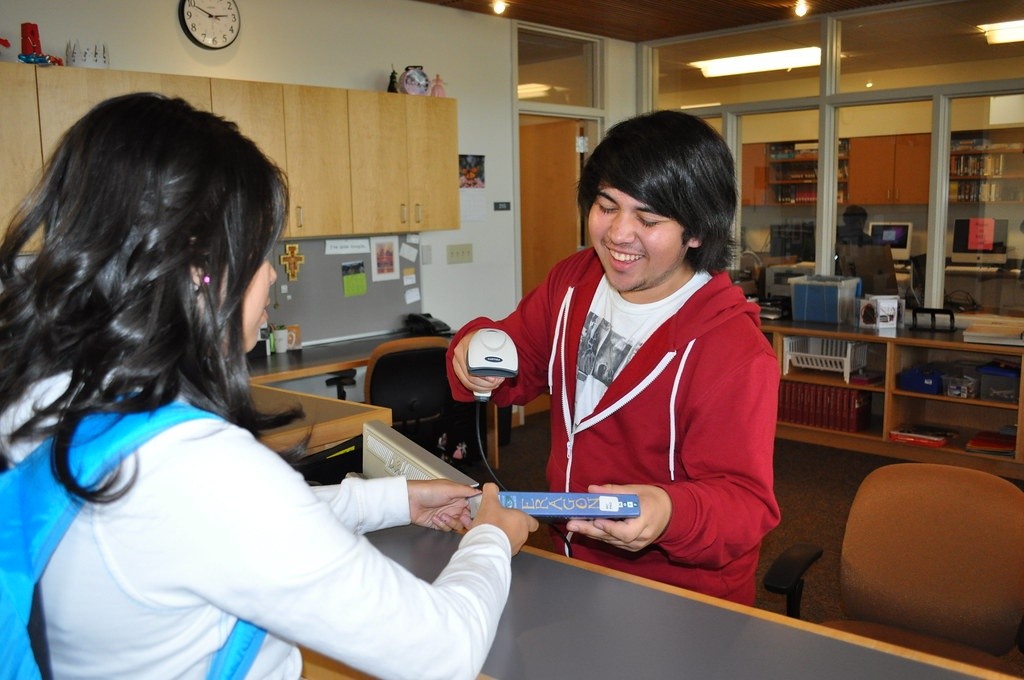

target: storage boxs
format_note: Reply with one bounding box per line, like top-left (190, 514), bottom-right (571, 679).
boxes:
top-left (770, 153), bottom-right (793, 159)
top-left (941, 371), bottom-right (979, 401)
top-left (978, 367), bottom-right (1018, 404)
top-left (899, 361), bottom-right (951, 394)
top-left (857, 298), bottom-right (900, 328)
top-left (949, 181), bottom-right (959, 200)
top-left (788, 276), bottom-right (860, 322)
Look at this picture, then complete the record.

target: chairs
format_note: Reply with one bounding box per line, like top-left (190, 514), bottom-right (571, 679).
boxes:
top-left (361, 333), bottom-right (463, 445)
top-left (761, 458), bottom-right (1024, 676)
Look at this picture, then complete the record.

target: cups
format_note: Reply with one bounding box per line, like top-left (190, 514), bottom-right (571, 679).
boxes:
top-left (273, 330), bottom-right (296, 354)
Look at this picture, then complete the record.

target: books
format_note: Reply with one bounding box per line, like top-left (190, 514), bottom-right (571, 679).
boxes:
top-left (966, 429), bottom-right (1017, 459)
top-left (778, 380), bottom-right (872, 433)
top-left (890, 420), bottom-right (958, 447)
top-left (963, 321), bottom-right (1024, 347)
top-left (949, 139), bottom-right (1021, 201)
top-left (469, 490), bottom-right (641, 520)
top-left (771, 140), bottom-right (849, 201)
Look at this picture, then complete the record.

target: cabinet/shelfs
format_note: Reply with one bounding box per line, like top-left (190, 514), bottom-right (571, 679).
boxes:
top-left (0, 53), bottom-right (50, 259)
top-left (37, 54), bottom-right (210, 178)
top-left (849, 135), bottom-right (929, 206)
top-left (950, 129), bottom-right (1023, 205)
top-left (757, 297), bottom-right (1024, 479)
top-left (349, 85), bottom-right (463, 241)
top-left (740, 139), bottom-right (764, 206)
top-left (766, 139), bottom-right (846, 205)
top-left (211, 72), bottom-right (351, 247)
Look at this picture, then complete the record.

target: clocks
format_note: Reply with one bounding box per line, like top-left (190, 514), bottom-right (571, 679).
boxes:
top-left (178, 0), bottom-right (240, 49)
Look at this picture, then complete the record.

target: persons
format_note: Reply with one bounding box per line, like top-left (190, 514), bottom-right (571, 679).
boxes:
top-left (446, 110), bottom-right (782, 609)
top-left (429, 72), bottom-right (448, 96)
top-left (0, 91), bottom-right (538, 680)
top-left (836, 205), bottom-right (872, 247)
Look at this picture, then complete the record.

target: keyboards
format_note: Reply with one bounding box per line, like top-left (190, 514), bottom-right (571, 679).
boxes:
top-left (894, 264), bottom-right (905, 270)
top-left (946, 266), bottom-right (999, 272)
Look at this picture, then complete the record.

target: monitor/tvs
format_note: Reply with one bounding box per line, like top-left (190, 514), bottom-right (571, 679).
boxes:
top-left (951, 219), bottom-right (1008, 265)
top-left (869, 222), bottom-right (912, 260)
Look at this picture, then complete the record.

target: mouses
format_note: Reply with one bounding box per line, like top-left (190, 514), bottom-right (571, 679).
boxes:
top-left (1011, 268), bottom-right (1021, 273)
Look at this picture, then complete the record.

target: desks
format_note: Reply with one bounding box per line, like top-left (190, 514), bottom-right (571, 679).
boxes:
top-left (245, 331), bottom-right (459, 384)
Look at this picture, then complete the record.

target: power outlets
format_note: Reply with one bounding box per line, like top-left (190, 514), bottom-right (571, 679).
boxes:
top-left (448, 243), bottom-right (473, 264)
